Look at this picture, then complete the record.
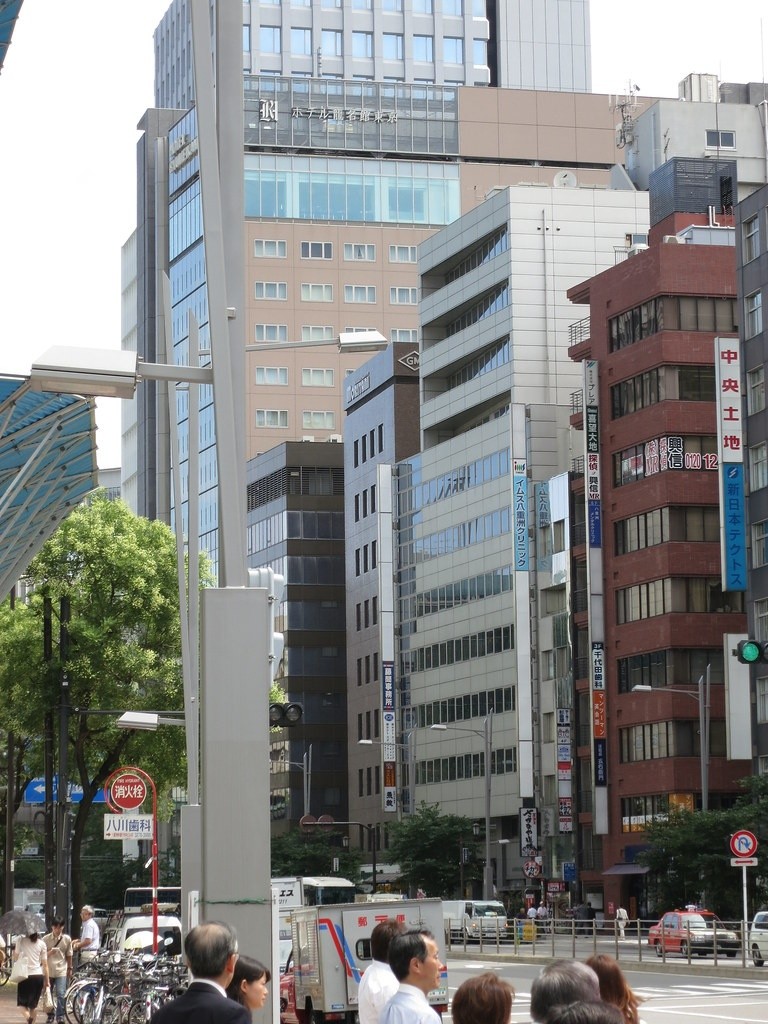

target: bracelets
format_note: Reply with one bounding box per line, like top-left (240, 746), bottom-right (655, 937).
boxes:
top-left (68, 967), bottom-right (73, 969)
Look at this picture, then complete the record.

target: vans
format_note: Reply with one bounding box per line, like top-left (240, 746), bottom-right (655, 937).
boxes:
top-left (748, 911), bottom-right (768, 967)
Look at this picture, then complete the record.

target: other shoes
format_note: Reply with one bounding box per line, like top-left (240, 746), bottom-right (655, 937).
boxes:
top-left (57, 1017), bottom-right (65, 1024)
top-left (28, 1016), bottom-right (34, 1024)
top-left (46, 1017), bottom-right (54, 1023)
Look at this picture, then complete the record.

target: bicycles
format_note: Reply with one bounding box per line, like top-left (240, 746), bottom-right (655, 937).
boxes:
top-left (63, 928), bottom-right (189, 1023)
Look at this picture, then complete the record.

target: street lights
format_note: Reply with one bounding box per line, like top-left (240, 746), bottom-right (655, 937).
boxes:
top-left (357, 730), bottom-right (414, 822)
top-left (431, 706), bottom-right (495, 899)
top-left (460, 838), bottom-right (510, 898)
top-left (183, 307), bottom-right (387, 934)
top-left (631, 663), bottom-right (712, 815)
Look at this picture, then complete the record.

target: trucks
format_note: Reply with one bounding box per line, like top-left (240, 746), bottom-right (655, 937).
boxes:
top-left (279, 897), bottom-right (449, 1024)
top-left (442, 901), bottom-right (509, 945)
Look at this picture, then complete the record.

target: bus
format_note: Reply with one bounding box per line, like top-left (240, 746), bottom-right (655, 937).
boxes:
top-left (123, 887), bottom-right (181, 913)
top-left (272, 877), bottom-right (355, 973)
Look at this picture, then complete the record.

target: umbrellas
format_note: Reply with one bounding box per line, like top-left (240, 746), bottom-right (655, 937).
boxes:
top-left (0, 910), bottom-right (47, 936)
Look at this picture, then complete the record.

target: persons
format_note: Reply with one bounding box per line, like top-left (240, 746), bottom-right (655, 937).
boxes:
top-left (616, 904), bottom-right (630, 940)
top-left (151, 920), bottom-right (252, 1024)
top-left (357, 920), bottom-right (403, 1024)
top-left (72, 904), bottom-right (100, 970)
top-left (575, 902), bottom-right (597, 935)
top-left (225, 955), bottom-right (270, 1013)
top-left (13, 932), bottom-right (50, 1024)
top-left (378, 928), bottom-right (444, 1024)
top-left (42, 915), bottom-right (73, 1024)
top-left (516, 901), bottom-right (548, 938)
top-left (530, 955), bottom-right (642, 1024)
top-left (452, 972), bottom-right (515, 1024)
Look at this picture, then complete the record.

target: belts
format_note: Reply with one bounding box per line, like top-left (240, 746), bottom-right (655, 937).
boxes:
top-left (82, 949), bottom-right (97, 951)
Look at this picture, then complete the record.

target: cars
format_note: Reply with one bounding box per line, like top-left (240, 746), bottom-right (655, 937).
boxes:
top-left (647, 905), bottom-right (740, 960)
top-left (25, 903), bottom-right (182, 970)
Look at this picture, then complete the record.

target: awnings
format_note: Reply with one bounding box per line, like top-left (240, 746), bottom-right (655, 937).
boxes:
top-left (602, 862), bottom-right (650, 874)
top-left (364, 872), bottom-right (409, 883)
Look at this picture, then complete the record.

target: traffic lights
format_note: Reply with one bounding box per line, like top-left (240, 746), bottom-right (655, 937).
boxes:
top-left (271, 703), bottom-right (305, 728)
top-left (735, 639), bottom-right (768, 664)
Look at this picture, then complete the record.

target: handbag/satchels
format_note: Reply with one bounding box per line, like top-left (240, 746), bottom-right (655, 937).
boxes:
top-left (617, 917), bottom-right (622, 922)
top-left (10, 956), bottom-right (29, 983)
top-left (43, 986), bottom-right (54, 1013)
